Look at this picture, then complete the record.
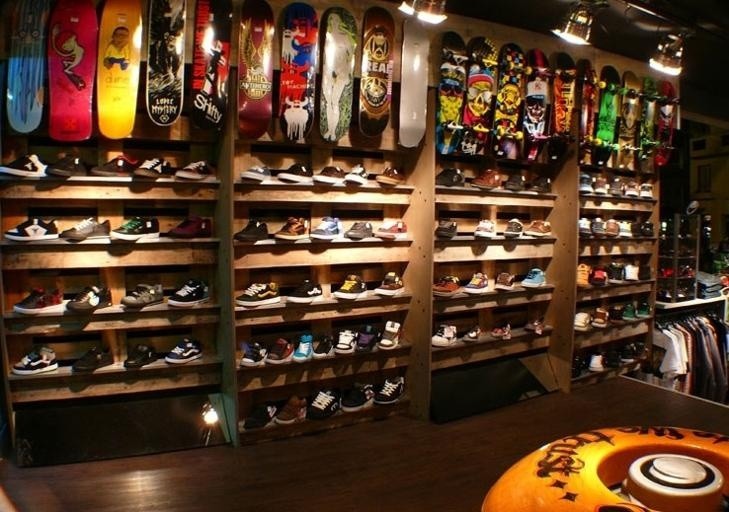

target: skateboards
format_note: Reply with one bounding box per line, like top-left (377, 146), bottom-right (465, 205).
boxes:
top-left (318, 6), bottom-right (365, 140)
top-left (272, 1), bottom-right (319, 148)
top-left (145, 1), bottom-right (187, 126)
top-left (359, 6), bottom-right (396, 137)
top-left (236, 1), bottom-right (275, 141)
top-left (96, 1), bottom-right (144, 139)
top-left (48, 1), bottom-right (100, 143)
top-left (397, 18), bottom-right (431, 147)
top-left (6, 1), bottom-right (48, 134)
top-left (189, 0), bottom-right (236, 131)
top-left (436, 31), bottom-right (680, 175)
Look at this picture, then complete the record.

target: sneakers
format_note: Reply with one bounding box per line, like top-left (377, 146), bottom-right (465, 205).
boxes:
top-left (572, 170), bottom-right (651, 379)
top-left (131, 157), bottom-right (172, 179)
top-left (90, 153), bottom-right (141, 179)
top-left (167, 214), bottom-right (213, 240)
top-left (119, 282), bottom-right (165, 309)
top-left (0, 154), bottom-right (47, 178)
top-left (4, 217), bottom-right (60, 242)
top-left (12, 347), bottom-right (59, 376)
top-left (109, 215), bottom-right (161, 241)
top-left (43, 152), bottom-right (88, 178)
top-left (66, 283), bottom-right (113, 312)
top-left (167, 276), bottom-right (211, 308)
top-left (234, 163), bottom-right (409, 432)
top-left (123, 340), bottom-right (158, 369)
top-left (58, 216), bottom-right (112, 242)
top-left (163, 337), bottom-right (203, 365)
top-left (12, 287), bottom-right (66, 315)
top-left (431, 164), bottom-right (554, 348)
top-left (174, 158), bottom-right (218, 182)
top-left (72, 343), bottom-right (114, 372)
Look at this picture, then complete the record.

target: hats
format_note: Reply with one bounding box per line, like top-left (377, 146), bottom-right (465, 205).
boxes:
top-left (525, 80), bottom-right (548, 105)
top-left (467, 62), bottom-right (496, 91)
top-left (441, 62), bottom-right (467, 87)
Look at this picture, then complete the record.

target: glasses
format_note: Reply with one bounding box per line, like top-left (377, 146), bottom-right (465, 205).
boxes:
top-left (439, 83), bottom-right (465, 97)
top-left (467, 87), bottom-right (493, 102)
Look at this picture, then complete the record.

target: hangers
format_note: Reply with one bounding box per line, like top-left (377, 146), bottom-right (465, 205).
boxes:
top-left (651, 304), bottom-right (729, 335)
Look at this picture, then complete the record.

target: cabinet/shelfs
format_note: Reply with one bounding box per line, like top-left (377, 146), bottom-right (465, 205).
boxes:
top-left (659, 212), bottom-right (710, 303)
top-left (0, 0), bottom-right (667, 471)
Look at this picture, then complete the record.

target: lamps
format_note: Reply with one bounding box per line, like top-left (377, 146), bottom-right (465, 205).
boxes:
top-left (398, 0), bottom-right (689, 79)
